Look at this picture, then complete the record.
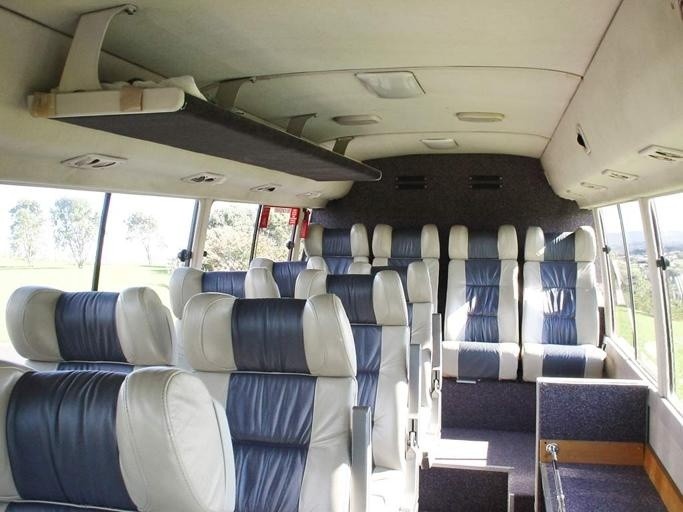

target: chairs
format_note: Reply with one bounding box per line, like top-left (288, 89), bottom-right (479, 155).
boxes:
top-left (442, 225), bottom-right (520, 383)
top-left (520, 225), bottom-right (608, 384)
